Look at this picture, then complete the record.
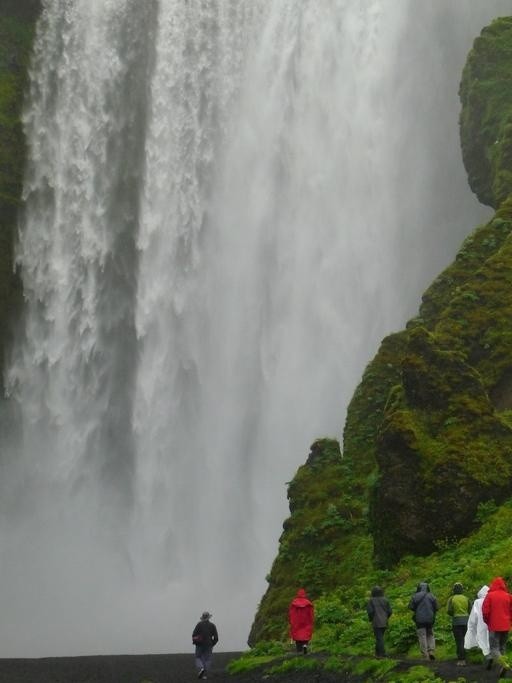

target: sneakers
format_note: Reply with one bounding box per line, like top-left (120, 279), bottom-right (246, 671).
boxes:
top-left (198, 667), bottom-right (204, 678)
top-left (429, 651), bottom-right (435, 660)
top-left (486, 656), bottom-right (493, 671)
top-left (303, 644), bottom-right (307, 654)
top-left (498, 664), bottom-right (509, 679)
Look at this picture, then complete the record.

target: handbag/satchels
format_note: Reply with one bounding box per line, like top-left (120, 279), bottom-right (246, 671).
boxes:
top-left (192, 635), bottom-right (203, 646)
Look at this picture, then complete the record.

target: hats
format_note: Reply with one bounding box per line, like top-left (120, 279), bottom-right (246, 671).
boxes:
top-left (200, 612), bottom-right (212, 621)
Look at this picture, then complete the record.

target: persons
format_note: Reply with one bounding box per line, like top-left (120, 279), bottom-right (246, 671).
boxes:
top-left (408, 582), bottom-right (441, 661)
top-left (464, 585), bottom-right (494, 670)
top-left (445, 582), bottom-right (473, 667)
top-left (366, 586), bottom-right (392, 657)
top-left (191, 611), bottom-right (219, 680)
top-left (288, 588), bottom-right (314, 654)
top-left (482, 576), bottom-right (512, 678)
